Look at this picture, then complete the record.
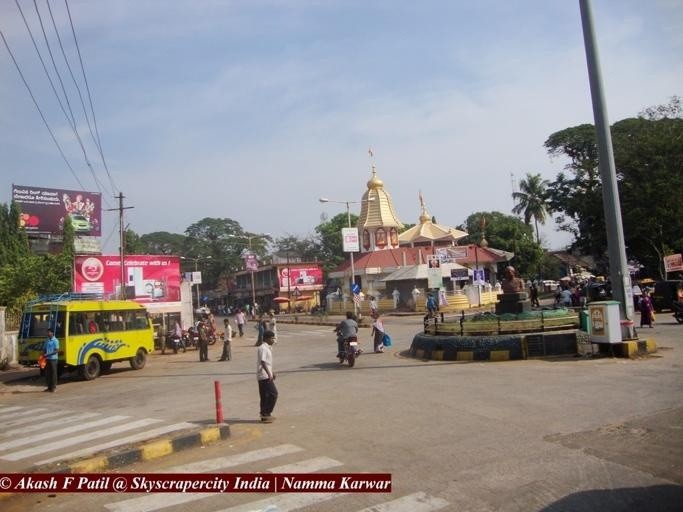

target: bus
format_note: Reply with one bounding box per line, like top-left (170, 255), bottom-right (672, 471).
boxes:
top-left (15, 292), bottom-right (155, 381)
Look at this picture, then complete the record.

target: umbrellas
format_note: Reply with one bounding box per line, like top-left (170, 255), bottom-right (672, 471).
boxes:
top-left (294, 295), bottom-right (313, 302)
top-left (272, 295), bottom-right (289, 305)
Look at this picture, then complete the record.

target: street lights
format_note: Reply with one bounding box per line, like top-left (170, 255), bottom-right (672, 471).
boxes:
top-left (317, 197), bottom-right (376, 316)
top-left (180, 255), bottom-right (211, 309)
top-left (227, 232), bottom-right (270, 318)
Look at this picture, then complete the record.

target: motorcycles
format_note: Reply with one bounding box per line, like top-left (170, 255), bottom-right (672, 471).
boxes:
top-left (165, 322), bottom-right (215, 354)
top-left (332, 323), bottom-right (357, 367)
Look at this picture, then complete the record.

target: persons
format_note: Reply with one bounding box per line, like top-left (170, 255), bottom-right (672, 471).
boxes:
top-left (498, 267), bottom-right (525, 293)
top-left (369, 313), bottom-right (388, 353)
top-left (528, 272), bottom-right (656, 329)
top-left (256, 330), bottom-right (277, 424)
top-left (157, 303), bottom-right (245, 363)
top-left (333, 312), bottom-right (358, 350)
top-left (267, 313), bottom-right (276, 338)
top-left (253, 314), bottom-right (269, 346)
top-left (424, 293), bottom-right (438, 317)
top-left (39, 328), bottom-right (59, 394)
top-left (368, 298), bottom-right (377, 316)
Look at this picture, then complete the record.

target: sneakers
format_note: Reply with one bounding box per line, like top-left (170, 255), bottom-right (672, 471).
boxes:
top-left (260, 416), bottom-right (276, 423)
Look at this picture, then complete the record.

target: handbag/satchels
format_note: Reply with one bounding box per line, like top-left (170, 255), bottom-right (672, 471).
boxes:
top-left (38, 355), bottom-right (47, 369)
top-left (649, 310), bottom-right (656, 321)
top-left (382, 333), bottom-right (392, 347)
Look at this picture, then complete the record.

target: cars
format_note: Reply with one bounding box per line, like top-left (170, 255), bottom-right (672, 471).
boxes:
top-left (66, 213), bottom-right (90, 233)
top-left (542, 259), bottom-right (683, 324)
